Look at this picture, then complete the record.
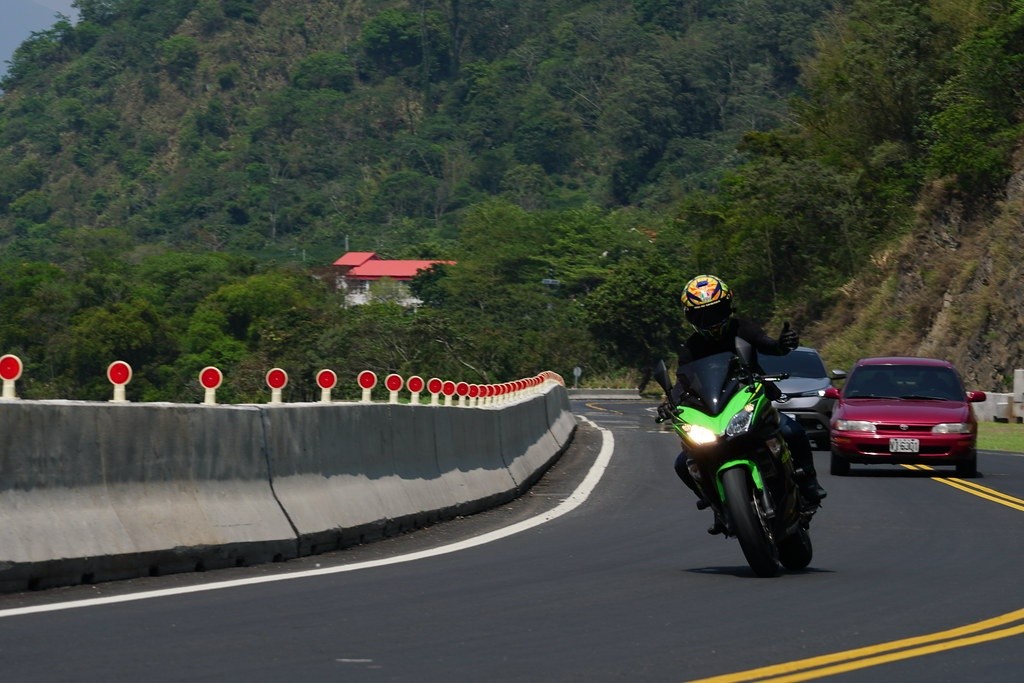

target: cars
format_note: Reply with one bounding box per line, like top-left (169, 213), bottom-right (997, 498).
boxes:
top-left (823, 357), bottom-right (986, 478)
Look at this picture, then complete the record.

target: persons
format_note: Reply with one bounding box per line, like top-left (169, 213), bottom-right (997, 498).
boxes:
top-left (657, 274), bottom-right (828, 536)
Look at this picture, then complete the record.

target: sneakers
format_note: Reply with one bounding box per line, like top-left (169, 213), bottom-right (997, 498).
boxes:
top-left (801, 480), bottom-right (827, 499)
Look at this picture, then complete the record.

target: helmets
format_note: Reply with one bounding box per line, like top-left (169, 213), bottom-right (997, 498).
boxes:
top-left (680, 275), bottom-right (737, 343)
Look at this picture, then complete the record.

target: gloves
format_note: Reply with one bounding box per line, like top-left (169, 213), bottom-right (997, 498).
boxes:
top-left (779, 321), bottom-right (799, 352)
top-left (657, 400), bottom-right (674, 421)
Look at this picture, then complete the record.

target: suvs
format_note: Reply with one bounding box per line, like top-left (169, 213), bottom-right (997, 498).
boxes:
top-left (753, 346), bottom-right (847, 451)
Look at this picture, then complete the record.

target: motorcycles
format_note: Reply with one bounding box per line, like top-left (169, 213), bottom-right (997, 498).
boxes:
top-left (654, 335), bottom-right (828, 577)
